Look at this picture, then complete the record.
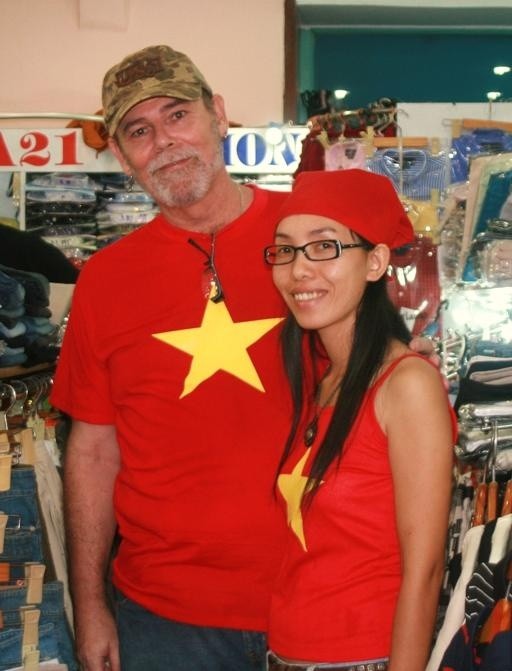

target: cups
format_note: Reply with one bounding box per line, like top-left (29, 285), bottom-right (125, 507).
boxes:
top-left (262, 235), bottom-right (370, 267)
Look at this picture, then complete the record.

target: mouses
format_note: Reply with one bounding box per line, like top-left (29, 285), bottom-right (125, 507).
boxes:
top-left (470, 419), bottom-right (511, 527)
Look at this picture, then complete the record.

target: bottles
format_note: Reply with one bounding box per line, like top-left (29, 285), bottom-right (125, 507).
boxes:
top-left (101, 45), bottom-right (214, 139)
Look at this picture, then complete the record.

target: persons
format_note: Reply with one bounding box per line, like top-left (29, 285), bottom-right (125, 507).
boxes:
top-left (49, 43), bottom-right (296, 671)
top-left (264, 167), bottom-right (461, 670)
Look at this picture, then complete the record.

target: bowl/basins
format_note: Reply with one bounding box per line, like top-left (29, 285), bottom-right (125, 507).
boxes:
top-left (232, 180), bottom-right (245, 219)
top-left (302, 362), bottom-right (345, 450)
